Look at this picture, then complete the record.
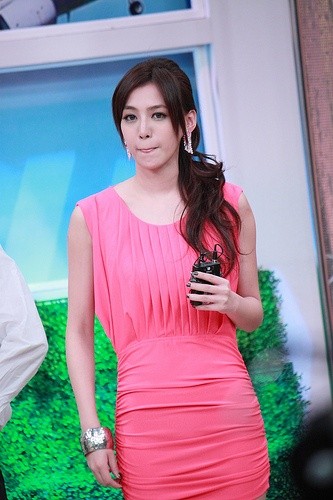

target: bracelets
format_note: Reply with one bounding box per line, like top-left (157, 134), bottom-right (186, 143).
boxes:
top-left (79, 427), bottom-right (114, 457)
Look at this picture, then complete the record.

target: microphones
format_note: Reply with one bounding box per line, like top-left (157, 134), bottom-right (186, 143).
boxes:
top-left (187, 242), bottom-right (224, 308)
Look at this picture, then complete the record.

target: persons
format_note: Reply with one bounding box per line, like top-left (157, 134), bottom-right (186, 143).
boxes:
top-left (0, 241), bottom-right (50, 488)
top-left (65, 58), bottom-right (272, 499)
